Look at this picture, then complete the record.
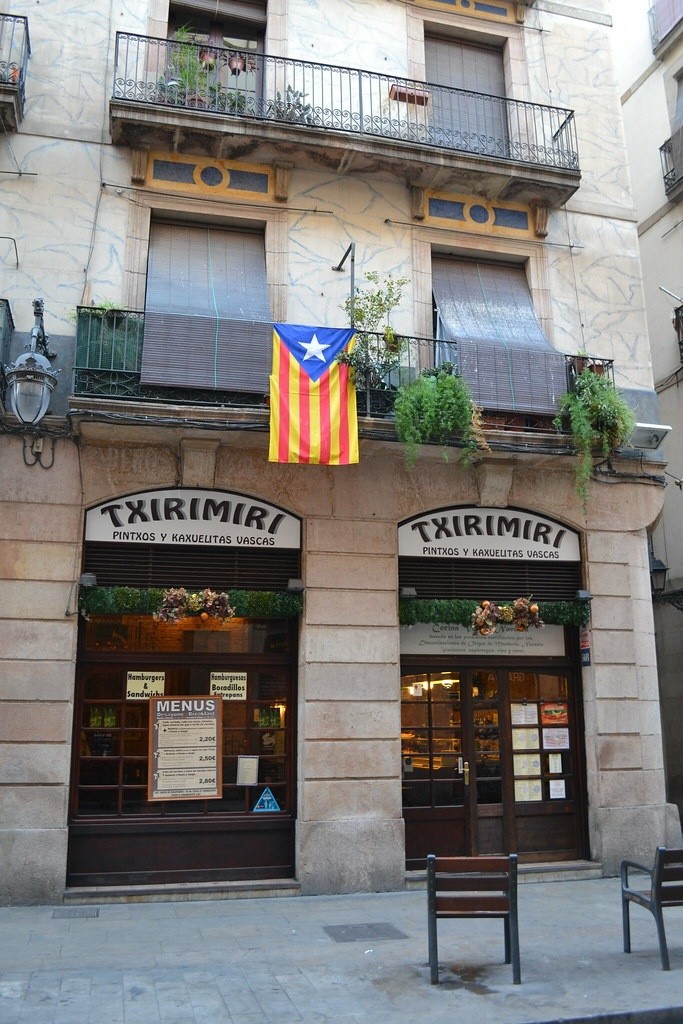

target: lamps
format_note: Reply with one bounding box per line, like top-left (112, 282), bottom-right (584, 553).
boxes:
top-left (400, 587), bottom-right (417, 599)
top-left (620, 423), bottom-right (673, 451)
top-left (287, 579), bottom-right (306, 592)
top-left (650, 551), bottom-right (670, 595)
top-left (77, 572), bottom-right (97, 588)
top-left (410, 672), bottom-right (453, 696)
top-left (3, 298), bottom-right (62, 427)
top-left (575, 590), bottom-right (594, 603)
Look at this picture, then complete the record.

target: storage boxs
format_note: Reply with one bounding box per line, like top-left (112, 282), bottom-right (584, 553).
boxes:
top-left (183, 629), bottom-right (231, 652)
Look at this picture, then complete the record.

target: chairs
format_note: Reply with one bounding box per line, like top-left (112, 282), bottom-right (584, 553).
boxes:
top-left (427, 853), bottom-right (522, 986)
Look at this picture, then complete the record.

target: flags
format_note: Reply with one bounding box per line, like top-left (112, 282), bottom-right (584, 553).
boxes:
top-left (268, 323), bottom-right (360, 465)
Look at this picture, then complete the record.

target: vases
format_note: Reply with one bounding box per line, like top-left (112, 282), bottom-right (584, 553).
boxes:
top-left (389, 84), bottom-right (429, 106)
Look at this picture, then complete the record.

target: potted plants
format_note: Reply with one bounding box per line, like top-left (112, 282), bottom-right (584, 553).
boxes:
top-left (198, 46), bottom-right (221, 71)
top-left (334, 271), bottom-right (411, 419)
top-left (221, 53), bottom-right (259, 77)
top-left (587, 362), bottom-right (605, 376)
top-left (571, 351), bottom-right (589, 375)
top-left (158, 84), bottom-right (185, 104)
top-left (185, 91), bottom-right (215, 108)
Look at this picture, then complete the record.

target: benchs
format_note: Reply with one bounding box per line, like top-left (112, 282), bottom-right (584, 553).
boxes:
top-left (621, 847), bottom-right (683, 973)
